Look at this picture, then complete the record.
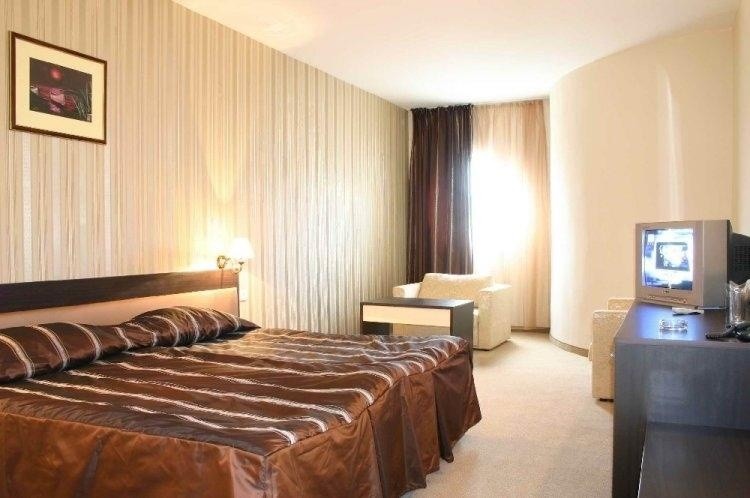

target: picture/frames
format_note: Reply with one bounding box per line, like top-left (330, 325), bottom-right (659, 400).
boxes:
top-left (8, 30), bottom-right (108, 145)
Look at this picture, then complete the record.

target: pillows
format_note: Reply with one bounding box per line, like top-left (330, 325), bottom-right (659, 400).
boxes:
top-left (0, 305), bottom-right (260, 386)
top-left (418, 273), bottom-right (486, 308)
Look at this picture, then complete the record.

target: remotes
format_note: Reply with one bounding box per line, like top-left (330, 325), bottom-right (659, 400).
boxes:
top-left (672, 307), bottom-right (704, 315)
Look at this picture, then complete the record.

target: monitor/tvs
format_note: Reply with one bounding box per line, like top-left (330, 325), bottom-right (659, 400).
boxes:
top-left (635, 220), bottom-right (750, 309)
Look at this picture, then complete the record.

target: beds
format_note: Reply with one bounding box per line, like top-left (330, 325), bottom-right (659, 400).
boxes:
top-left (1, 269), bottom-right (482, 498)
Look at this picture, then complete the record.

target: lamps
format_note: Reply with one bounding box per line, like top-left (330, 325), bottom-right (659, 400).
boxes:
top-left (217, 236), bottom-right (256, 274)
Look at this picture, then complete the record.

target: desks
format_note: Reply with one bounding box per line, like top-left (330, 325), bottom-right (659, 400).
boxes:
top-left (611, 300), bottom-right (750, 498)
top-left (358, 295), bottom-right (475, 372)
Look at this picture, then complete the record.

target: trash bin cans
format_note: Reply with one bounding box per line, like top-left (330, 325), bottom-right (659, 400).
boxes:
top-left (726, 321), bottom-right (750, 341)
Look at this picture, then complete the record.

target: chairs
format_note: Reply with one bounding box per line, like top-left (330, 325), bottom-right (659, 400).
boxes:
top-left (588, 297), bottom-right (635, 402)
top-left (391, 271), bottom-right (513, 351)
